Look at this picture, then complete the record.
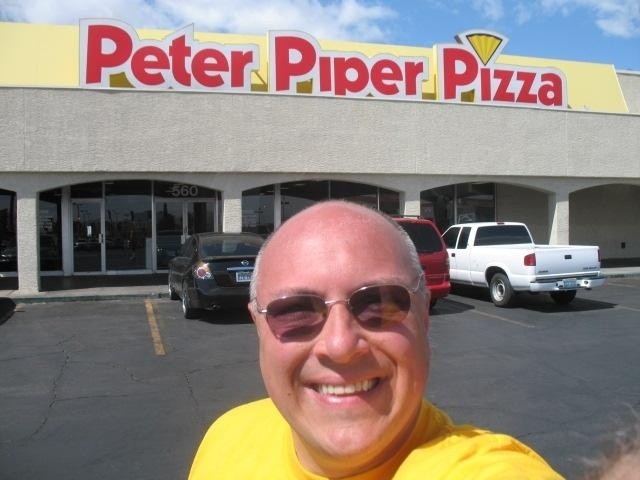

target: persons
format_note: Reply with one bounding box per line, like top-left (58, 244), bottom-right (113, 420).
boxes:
top-left (185, 199), bottom-right (640, 480)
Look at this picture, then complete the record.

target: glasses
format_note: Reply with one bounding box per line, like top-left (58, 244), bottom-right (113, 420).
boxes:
top-left (256, 275), bottom-right (422, 339)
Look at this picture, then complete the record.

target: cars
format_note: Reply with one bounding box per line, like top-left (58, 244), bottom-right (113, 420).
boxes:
top-left (0, 235), bottom-right (57, 271)
top-left (168, 218), bottom-right (450, 322)
top-left (75, 238), bottom-right (101, 250)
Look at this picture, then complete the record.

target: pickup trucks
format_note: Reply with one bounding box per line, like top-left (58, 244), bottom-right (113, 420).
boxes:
top-left (440, 221), bottom-right (606, 308)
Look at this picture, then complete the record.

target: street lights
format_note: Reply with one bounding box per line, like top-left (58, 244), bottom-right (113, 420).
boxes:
top-left (281, 201), bottom-right (290, 223)
top-left (71, 202), bottom-right (84, 220)
top-left (253, 209), bottom-right (264, 224)
